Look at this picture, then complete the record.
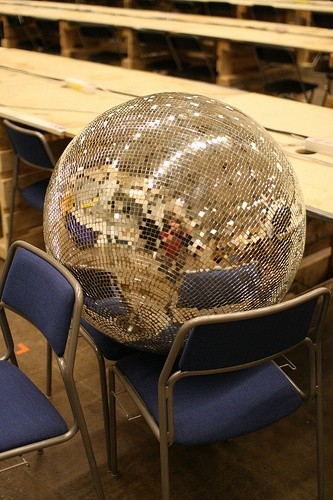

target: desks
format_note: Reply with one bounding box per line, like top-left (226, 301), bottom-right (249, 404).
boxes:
top-left (0, 0), bottom-right (333, 217)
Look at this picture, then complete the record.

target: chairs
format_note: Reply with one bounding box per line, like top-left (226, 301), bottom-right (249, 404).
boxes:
top-left (46, 318), bottom-right (145, 475)
top-left (3, 119), bottom-right (58, 247)
top-left (105, 288), bottom-right (333, 500)
top-left (0, 241), bottom-right (108, 500)
